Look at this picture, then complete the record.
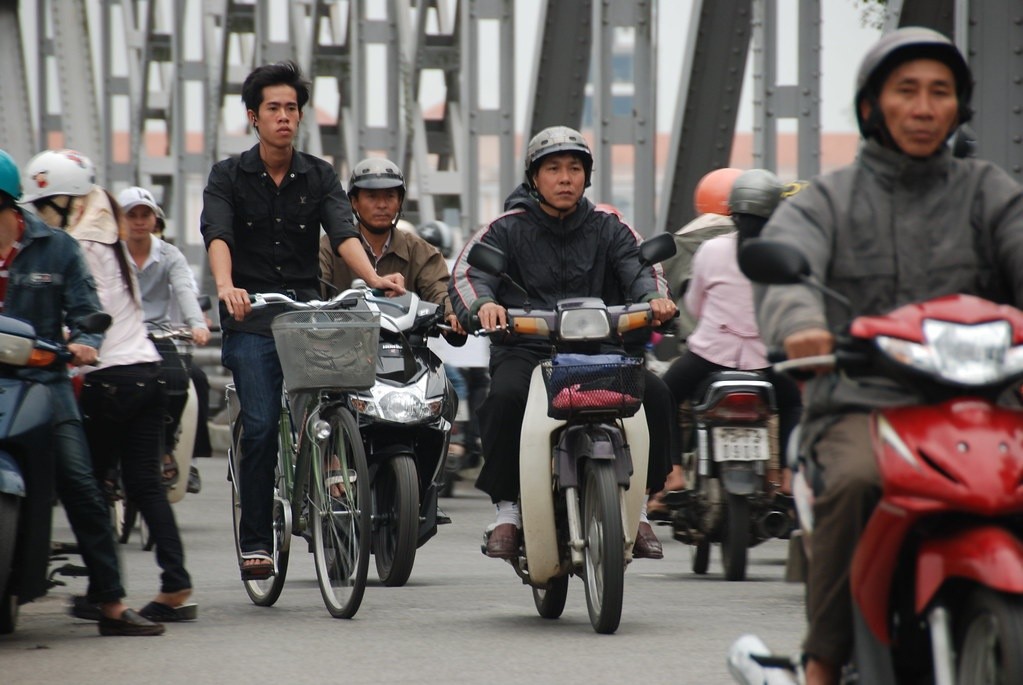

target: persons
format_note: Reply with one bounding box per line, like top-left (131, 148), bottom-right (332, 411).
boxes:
top-left (663, 163), bottom-right (803, 504)
top-left (752, 26), bottom-right (1023, 685)
top-left (20, 149), bottom-right (214, 624)
top-left (320, 153), bottom-right (488, 531)
top-left (200, 64), bottom-right (407, 581)
top-left (0, 148), bottom-right (168, 638)
top-left (446, 125), bottom-right (677, 559)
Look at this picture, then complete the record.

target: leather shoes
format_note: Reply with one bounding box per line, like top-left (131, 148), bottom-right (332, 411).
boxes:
top-left (99, 609), bottom-right (165, 635)
top-left (486, 523), bottom-right (520, 558)
top-left (633, 521), bottom-right (664, 559)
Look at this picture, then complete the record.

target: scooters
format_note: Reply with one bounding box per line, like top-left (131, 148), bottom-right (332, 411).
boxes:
top-left (113, 320), bottom-right (200, 551)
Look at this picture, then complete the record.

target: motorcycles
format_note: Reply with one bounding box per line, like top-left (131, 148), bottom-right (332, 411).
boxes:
top-left (725, 240), bottom-right (1022, 684)
top-left (658, 370), bottom-right (800, 582)
top-left (1, 312), bottom-right (115, 632)
top-left (319, 275), bottom-right (468, 589)
top-left (466, 233), bottom-right (681, 636)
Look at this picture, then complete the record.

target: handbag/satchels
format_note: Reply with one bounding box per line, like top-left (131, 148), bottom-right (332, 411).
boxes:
top-left (278, 315), bottom-right (380, 386)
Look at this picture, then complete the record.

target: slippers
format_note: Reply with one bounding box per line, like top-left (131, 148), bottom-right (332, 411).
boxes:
top-left (239, 551), bottom-right (273, 580)
top-left (66, 598), bottom-right (101, 620)
top-left (324, 466), bottom-right (357, 507)
top-left (138, 602), bottom-right (197, 621)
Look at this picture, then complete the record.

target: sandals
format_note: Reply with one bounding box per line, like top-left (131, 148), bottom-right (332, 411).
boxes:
top-left (655, 487), bottom-right (688, 499)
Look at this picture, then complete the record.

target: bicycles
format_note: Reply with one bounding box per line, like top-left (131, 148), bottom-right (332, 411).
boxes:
top-left (223, 278), bottom-right (387, 619)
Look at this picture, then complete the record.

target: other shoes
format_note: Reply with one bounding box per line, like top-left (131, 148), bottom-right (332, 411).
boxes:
top-left (646, 500), bottom-right (671, 518)
top-left (187, 467), bottom-right (201, 493)
top-left (161, 453), bottom-right (179, 486)
top-left (436, 506), bottom-right (451, 525)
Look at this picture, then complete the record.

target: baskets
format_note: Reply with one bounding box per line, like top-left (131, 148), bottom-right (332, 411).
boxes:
top-left (270, 310), bottom-right (381, 394)
top-left (540, 358), bottom-right (645, 417)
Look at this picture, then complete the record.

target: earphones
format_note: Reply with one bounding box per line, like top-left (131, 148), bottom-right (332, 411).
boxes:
top-left (297, 121), bottom-right (300, 127)
top-left (252, 115), bottom-right (256, 127)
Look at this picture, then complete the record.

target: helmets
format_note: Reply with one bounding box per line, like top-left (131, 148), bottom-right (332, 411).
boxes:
top-left (524, 125), bottom-right (593, 180)
top-left (391, 219), bottom-right (453, 258)
top-left (14, 150), bottom-right (92, 204)
top-left (694, 168), bottom-right (810, 220)
top-left (0, 150), bottom-right (20, 197)
top-left (854, 26), bottom-right (974, 140)
top-left (347, 157), bottom-right (406, 197)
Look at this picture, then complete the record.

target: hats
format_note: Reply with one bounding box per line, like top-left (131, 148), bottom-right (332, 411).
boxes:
top-left (118, 186), bottom-right (158, 215)
top-left (156, 205), bottom-right (166, 230)
